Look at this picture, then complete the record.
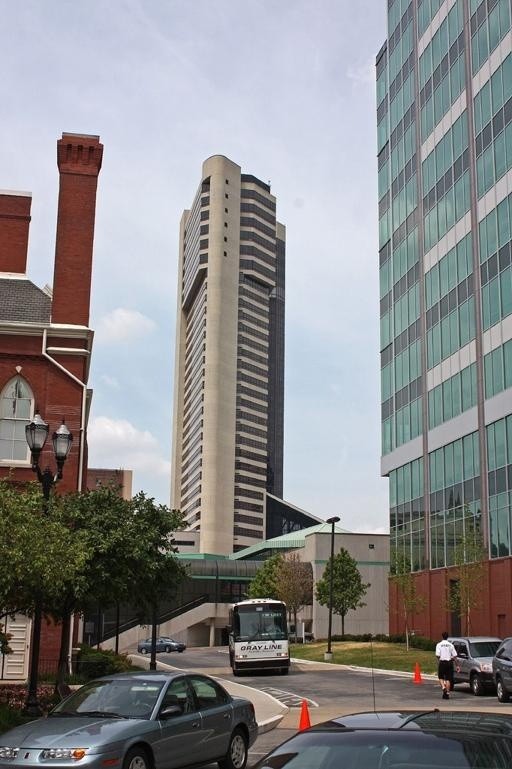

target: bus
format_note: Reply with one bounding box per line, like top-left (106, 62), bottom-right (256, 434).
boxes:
top-left (225, 597), bottom-right (294, 676)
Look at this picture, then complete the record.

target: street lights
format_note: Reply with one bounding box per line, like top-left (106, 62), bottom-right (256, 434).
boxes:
top-left (21, 408), bottom-right (74, 719)
top-left (324, 516), bottom-right (341, 662)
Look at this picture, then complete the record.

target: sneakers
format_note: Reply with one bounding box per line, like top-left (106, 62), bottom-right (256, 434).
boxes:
top-left (442, 687), bottom-right (449, 699)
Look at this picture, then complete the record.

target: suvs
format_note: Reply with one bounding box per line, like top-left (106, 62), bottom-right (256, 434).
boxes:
top-left (447, 635), bottom-right (512, 703)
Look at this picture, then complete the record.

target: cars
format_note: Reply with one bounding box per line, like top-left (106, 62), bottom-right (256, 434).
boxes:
top-left (249, 707), bottom-right (512, 769)
top-left (0, 669), bottom-right (259, 769)
top-left (138, 636), bottom-right (187, 653)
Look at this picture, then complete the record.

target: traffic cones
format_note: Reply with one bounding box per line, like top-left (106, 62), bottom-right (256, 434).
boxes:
top-left (413, 661), bottom-right (424, 685)
top-left (298, 699), bottom-right (311, 733)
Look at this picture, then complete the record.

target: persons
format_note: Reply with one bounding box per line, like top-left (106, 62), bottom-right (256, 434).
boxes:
top-left (435, 631), bottom-right (461, 699)
